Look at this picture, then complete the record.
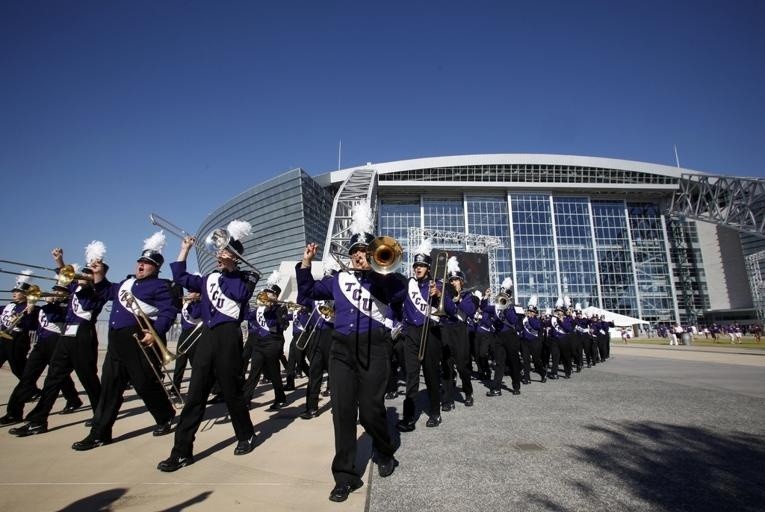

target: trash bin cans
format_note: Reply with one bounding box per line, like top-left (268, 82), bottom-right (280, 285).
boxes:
top-left (681, 332), bottom-right (690, 345)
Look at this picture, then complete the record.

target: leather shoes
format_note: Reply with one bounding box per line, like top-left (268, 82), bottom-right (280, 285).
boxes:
top-left (542, 357), bottom-right (604, 383)
top-left (386, 377), bottom-right (531, 432)
top-left (72, 433), bottom-right (112, 450)
top-left (271, 383), bottom-right (332, 419)
top-left (59, 400), bottom-right (82, 414)
top-left (157, 454), bottom-right (194, 472)
top-left (25, 388), bottom-right (42, 404)
top-left (330, 480), bottom-right (350, 502)
top-left (153, 413), bottom-right (175, 436)
top-left (378, 456), bottom-right (394, 477)
top-left (234, 434), bottom-right (257, 455)
top-left (0, 414), bottom-right (48, 436)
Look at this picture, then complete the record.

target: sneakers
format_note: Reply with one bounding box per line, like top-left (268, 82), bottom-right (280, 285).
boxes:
top-left (207, 397), bottom-right (224, 404)
top-left (166, 389), bottom-right (180, 396)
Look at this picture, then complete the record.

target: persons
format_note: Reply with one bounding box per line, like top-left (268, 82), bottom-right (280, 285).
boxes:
top-left (0, 219), bottom-right (615, 472)
top-left (296, 201), bottom-right (403, 502)
top-left (621, 321), bottom-right (762, 345)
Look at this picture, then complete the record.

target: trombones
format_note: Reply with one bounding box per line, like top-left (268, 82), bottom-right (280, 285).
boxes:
top-left (149, 212), bottom-right (263, 280)
top-left (1, 284), bottom-right (71, 304)
top-left (177, 319), bottom-right (202, 354)
top-left (257, 291), bottom-right (302, 312)
top-left (417, 252), bottom-right (449, 361)
top-left (494, 292), bottom-right (510, 311)
top-left (295, 298), bottom-right (336, 350)
top-left (1, 310), bottom-right (27, 340)
top-left (124, 290), bottom-right (186, 409)
top-left (1, 260), bottom-right (93, 286)
top-left (331, 236), bottom-right (403, 273)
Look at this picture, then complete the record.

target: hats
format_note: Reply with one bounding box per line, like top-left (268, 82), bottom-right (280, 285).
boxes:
top-left (52, 282), bottom-right (70, 291)
top-left (82, 260), bottom-right (108, 276)
top-left (11, 282), bottom-right (30, 294)
top-left (447, 272), bottom-right (464, 283)
top-left (501, 287), bottom-right (511, 298)
top-left (265, 285), bottom-right (280, 294)
top-left (412, 254), bottom-right (431, 267)
top-left (527, 304), bottom-right (538, 313)
top-left (554, 306), bottom-right (605, 322)
top-left (349, 232), bottom-right (374, 253)
top-left (137, 250), bottom-right (164, 269)
top-left (225, 237), bottom-right (244, 256)
